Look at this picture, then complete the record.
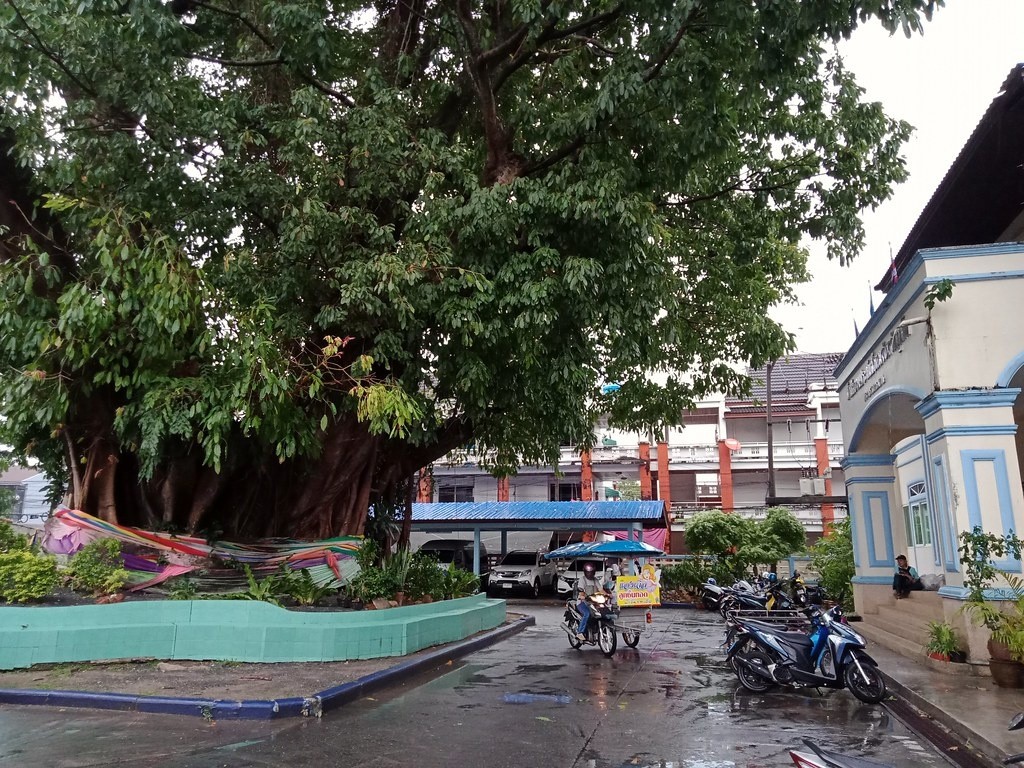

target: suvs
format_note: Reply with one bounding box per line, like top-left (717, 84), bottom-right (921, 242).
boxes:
top-left (487, 549), bottom-right (559, 599)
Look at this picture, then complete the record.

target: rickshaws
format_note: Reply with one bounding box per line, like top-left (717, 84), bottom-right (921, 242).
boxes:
top-left (560, 584), bottom-right (654, 657)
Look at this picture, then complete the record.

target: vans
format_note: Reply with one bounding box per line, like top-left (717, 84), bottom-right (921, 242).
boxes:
top-left (414, 539), bottom-right (489, 575)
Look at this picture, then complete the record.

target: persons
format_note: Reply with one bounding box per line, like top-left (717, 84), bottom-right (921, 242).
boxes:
top-left (575, 563), bottom-right (613, 641)
top-left (611, 561), bottom-right (621, 581)
top-left (619, 557), bottom-right (624, 576)
top-left (892, 555), bottom-right (922, 598)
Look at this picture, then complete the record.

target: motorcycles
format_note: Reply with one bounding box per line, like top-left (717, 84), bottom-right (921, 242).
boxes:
top-left (700, 569), bottom-right (886, 704)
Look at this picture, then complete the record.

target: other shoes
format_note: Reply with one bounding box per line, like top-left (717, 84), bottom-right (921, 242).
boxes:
top-left (576, 632), bottom-right (586, 641)
top-left (893, 591), bottom-right (902, 599)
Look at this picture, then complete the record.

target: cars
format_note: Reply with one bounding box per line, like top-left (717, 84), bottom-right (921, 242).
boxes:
top-left (558, 555), bottom-right (611, 600)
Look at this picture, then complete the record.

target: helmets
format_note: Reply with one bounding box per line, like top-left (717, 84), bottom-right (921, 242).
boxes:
top-left (583, 562), bottom-right (596, 579)
top-left (769, 573), bottom-right (777, 583)
top-left (763, 572), bottom-right (770, 579)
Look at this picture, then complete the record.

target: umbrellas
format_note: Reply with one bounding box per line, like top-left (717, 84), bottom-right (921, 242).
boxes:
top-left (543, 538), bottom-right (665, 583)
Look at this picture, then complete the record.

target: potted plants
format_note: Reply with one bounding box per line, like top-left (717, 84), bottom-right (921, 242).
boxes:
top-left (921, 619), bottom-right (966, 662)
top-left (957, 524), bottom-right (1024, 688)
top-left (422, 546), bottom-right (437, 603)
top-left (392, 540), bottom-right (411, 605)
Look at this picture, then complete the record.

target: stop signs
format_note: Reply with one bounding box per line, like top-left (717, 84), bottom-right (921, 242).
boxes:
top-left (725, 438), bottom-right (741, 450)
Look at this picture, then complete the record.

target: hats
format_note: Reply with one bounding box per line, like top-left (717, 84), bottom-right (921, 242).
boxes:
top-left (896, 554), bottom-right (906, 560)
